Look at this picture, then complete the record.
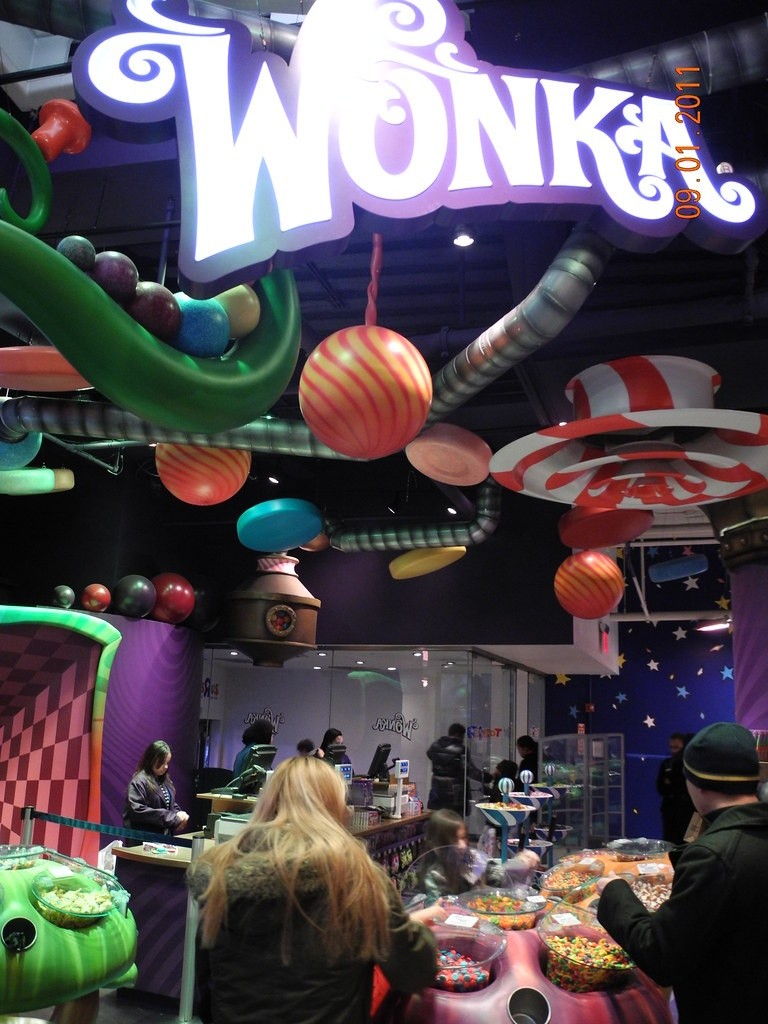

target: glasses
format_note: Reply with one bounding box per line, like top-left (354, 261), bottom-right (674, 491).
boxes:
top-left (272, 731), bottom-right (277, 736)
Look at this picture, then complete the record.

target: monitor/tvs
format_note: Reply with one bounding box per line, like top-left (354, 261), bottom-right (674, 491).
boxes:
top-left (366, 744), bottom-right (392, 778)
top-left (324, 743), bottom-right (348, 766)
top-left (239, 742), bottom-right (279, 796)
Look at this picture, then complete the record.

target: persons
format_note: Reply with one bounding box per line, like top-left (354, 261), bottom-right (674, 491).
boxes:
top-left (123, 741), bottom-right (188, 848)
top-left (296, 729), bottom-right (354, 774)
top-left (184, 757), bottom-right (436, 1024)
top-left (234, 720), bottom-right (274, 779)
top-left (480, 735), bottom-right (545, 803)
top-left (597, 723), bottom-right (768, 1024)
top-left (657, 734), bottom-right (695, 844)
top-left (426, 724), bottom-right (496, 817)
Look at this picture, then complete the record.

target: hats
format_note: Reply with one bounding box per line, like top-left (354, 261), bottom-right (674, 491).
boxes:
top-left (682, 721), bottom-right (761, 794)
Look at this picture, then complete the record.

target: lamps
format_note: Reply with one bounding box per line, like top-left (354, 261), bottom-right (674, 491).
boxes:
top-left (698, 617), bottom-right (731, 631)
top-left (450, 224), bottom-right (478, 250)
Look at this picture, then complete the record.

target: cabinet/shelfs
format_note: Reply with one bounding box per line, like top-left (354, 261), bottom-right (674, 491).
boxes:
top-left (536, 733), bottom-right (628, 855)
